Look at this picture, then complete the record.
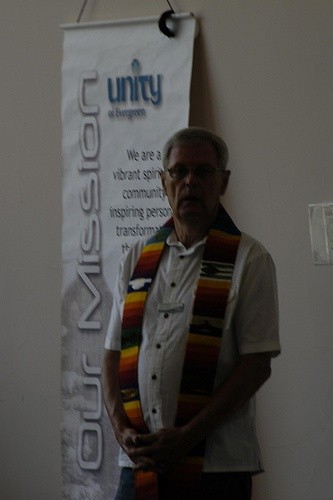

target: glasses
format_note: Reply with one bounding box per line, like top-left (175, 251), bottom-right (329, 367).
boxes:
top-left (164, 161), bottom-right (225, 181)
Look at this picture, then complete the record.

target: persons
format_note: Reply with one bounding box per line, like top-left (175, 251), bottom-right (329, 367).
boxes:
top-left (102, 127), bottom-right (281, 500)
